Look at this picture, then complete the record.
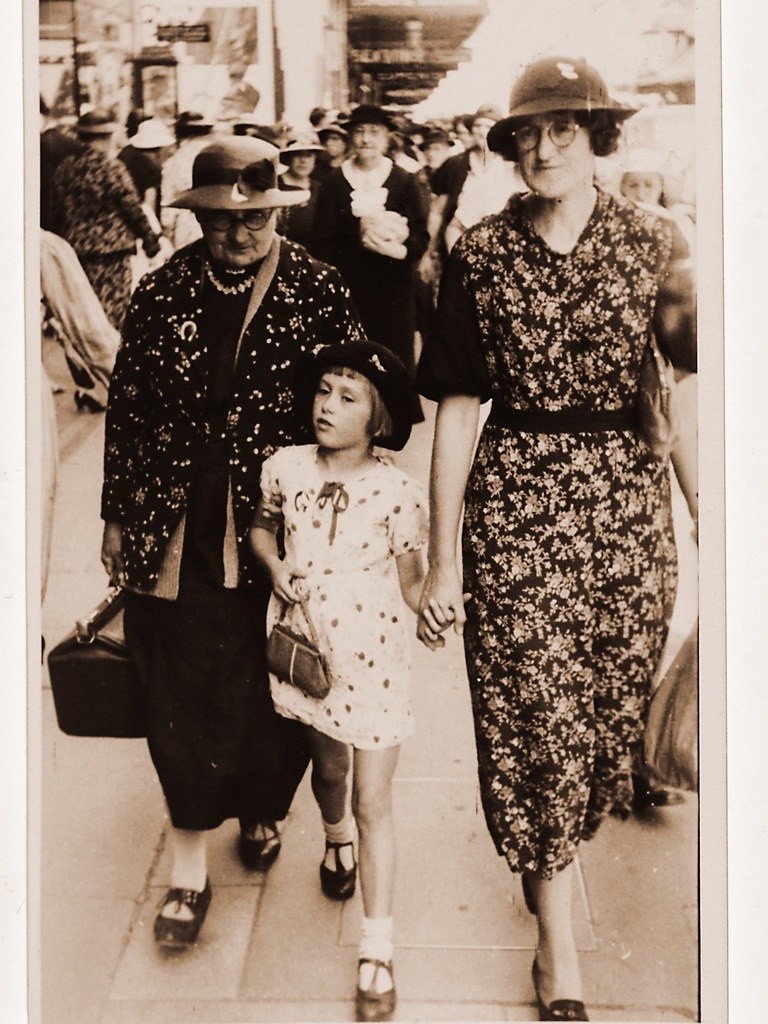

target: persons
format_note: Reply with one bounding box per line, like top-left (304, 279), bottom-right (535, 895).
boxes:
top-left (308, 106), bottom-right (432, 358)
top-left (415, 54), bottom-right (696, 1021)
top-left (39, 90), bottom-right (214, 416)
top-left (102, 136), bottom-right (369, 947)
top-left (233, 104), bottom-right (697, 281)
top-left (41, 222), bottom-right (122, 676)
top-left (248, 339), bottom-right (471, 1022)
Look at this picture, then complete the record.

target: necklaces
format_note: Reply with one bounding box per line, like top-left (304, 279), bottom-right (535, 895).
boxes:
top-left (203, 259), bottom-right (256, 294)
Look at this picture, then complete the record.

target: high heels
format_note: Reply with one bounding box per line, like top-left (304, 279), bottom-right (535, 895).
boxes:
top-left (73, 389), bottom-right (110, 412)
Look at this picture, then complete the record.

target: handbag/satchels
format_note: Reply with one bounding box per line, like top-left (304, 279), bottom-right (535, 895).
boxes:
top-left (45, 586), bottom-right (150, 739)
top-left (264, 597), bottom-right (333, 702)
top-left (640, 327), bottom-right (680, 454)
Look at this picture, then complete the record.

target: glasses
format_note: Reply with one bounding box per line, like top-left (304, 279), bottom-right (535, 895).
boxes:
top-left (196, 210), bottom-right (280, 232)
top-left (510, 121), bottom-right (594, 151)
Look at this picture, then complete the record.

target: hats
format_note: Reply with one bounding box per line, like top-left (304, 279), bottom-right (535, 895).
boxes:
top-left (419, 129), bottom-right (455, 152)
top-left (126, 107), bottom-right (153, 137)
top-left (317, 123), bottom-right (353, 153)
top-left (340, 104), bottom-right (399, 132)
top-left (159, 135), bottom-right (312, 212)
top-left (463, 104), bottom-right (504, 134)
top-left (130, 120), bottom-right (176, 149)
top-left (313, 341), bottom-right (426, 453)
top-left (280, 134), bottom-right (325, 165)
top-left (70, 109), bottom-right (117, 133)
top-left (485, 55), bottom-right (640, 163)
top-left (179, 111), bottom-right (212, 129)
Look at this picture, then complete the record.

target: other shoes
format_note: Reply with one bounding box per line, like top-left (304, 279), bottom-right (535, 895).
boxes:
top-left (531, 951), bottom-right (592, 1021)
top-left (354, 954), bottom-right (398, 1023)
top-left (238, 822), bottom-right (284, 871)
top-left (152, 875), bottom-right (212, 946)
top-left (318, 836), bottom-right (358, 902)
